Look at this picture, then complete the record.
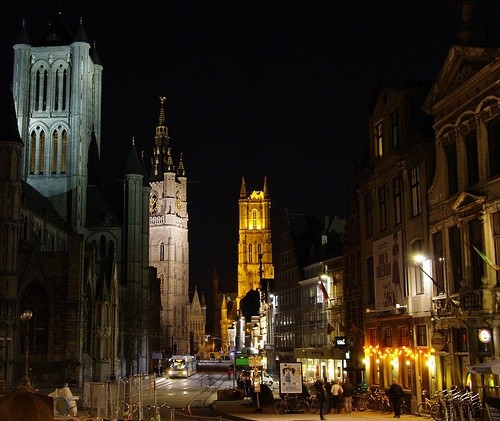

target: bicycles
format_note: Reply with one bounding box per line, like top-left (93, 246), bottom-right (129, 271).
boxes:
top-left (417, 386), bottom-right (484, 421)
top-left (274, 394), bottom-right (318, 414)
top-left (351, 387), bottom-right (408, 415)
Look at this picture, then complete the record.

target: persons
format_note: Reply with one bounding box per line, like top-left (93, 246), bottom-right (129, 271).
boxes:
top-left (228, 368), bottom-right (231, 380)
top-left (237, 376), bottom-right (249, 396)
top-left (390, 233), bottom-right (404, 305)
top-left (154, 365), bottom-right (163, 377)
top-left (314, 378), bottom-right (326, 420)
top-left (323, 377), bottom-right (353, 414)
top-left (387, 381), bottom-right (403, 418)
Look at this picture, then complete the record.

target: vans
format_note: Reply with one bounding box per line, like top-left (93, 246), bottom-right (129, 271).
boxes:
top-left (239, 370), bottom-right (273, 388)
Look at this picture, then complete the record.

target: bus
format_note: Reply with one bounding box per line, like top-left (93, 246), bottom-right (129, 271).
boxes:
top-left (168, 355), bottom-right (198, 378)
top-left (235, 352), bottom-right (264, 370)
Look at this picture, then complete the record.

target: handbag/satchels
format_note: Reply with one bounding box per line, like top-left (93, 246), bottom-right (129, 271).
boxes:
top-left (338, 385), bottom-right (343, 397)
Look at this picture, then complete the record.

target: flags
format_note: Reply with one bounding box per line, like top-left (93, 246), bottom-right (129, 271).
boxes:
top-left (327, 324), bottom-right (334, 335)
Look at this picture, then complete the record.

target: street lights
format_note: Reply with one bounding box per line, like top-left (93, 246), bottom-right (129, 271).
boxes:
top-left (20, 308), bottom-right (34, 393)
